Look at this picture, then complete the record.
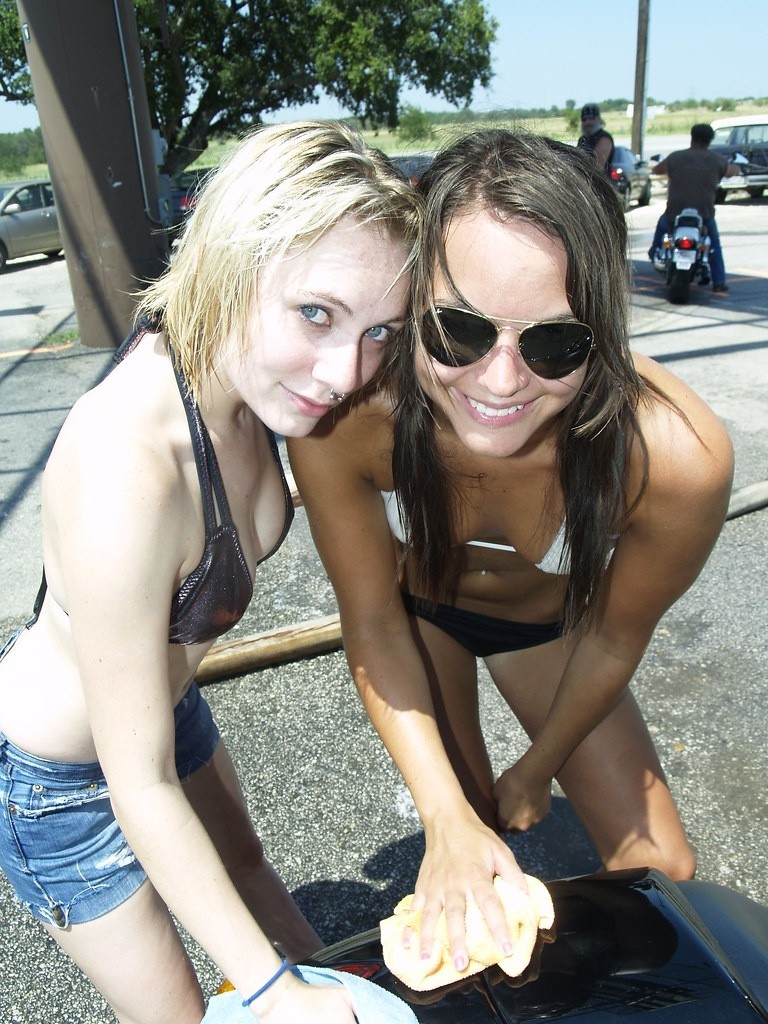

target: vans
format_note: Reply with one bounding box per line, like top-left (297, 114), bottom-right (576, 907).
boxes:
top-left (707, 115), bottom-right (768, 205)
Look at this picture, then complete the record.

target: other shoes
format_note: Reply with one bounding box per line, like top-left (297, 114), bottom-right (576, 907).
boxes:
top-left (713, 282), bottom-right (729, 294)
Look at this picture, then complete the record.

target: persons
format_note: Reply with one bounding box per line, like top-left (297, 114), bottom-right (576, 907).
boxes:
top-left (575, 103), bottom-right (615, 175)
top-left (0, 121), bottom-right (429, 1024)
top-left (285, 128), bottom-right (733, 977)
top-left (647, 124), bottom-right (741, 293)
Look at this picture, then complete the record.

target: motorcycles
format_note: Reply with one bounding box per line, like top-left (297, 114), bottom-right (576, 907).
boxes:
top-left (650, 155), bottom-right (749, 305)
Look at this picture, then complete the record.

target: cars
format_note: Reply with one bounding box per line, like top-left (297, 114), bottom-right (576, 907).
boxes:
top-left (607, 145), bottom-right (653, 211)
top-left (388, 155), bottom-right (438, 191)
top-left (0, 178), bottom-right (64, 270)
top-left (166, 167), bottom-right (218, 250)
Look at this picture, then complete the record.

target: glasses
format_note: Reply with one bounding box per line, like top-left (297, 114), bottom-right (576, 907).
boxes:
top-left (581, 115), bottom-right (596, 121)
top-left (419, 305), bottom-right (598, 380)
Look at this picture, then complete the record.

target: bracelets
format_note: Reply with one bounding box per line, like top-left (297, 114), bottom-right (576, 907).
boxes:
top-left (241, 960), bottom-right (287, 1008)
